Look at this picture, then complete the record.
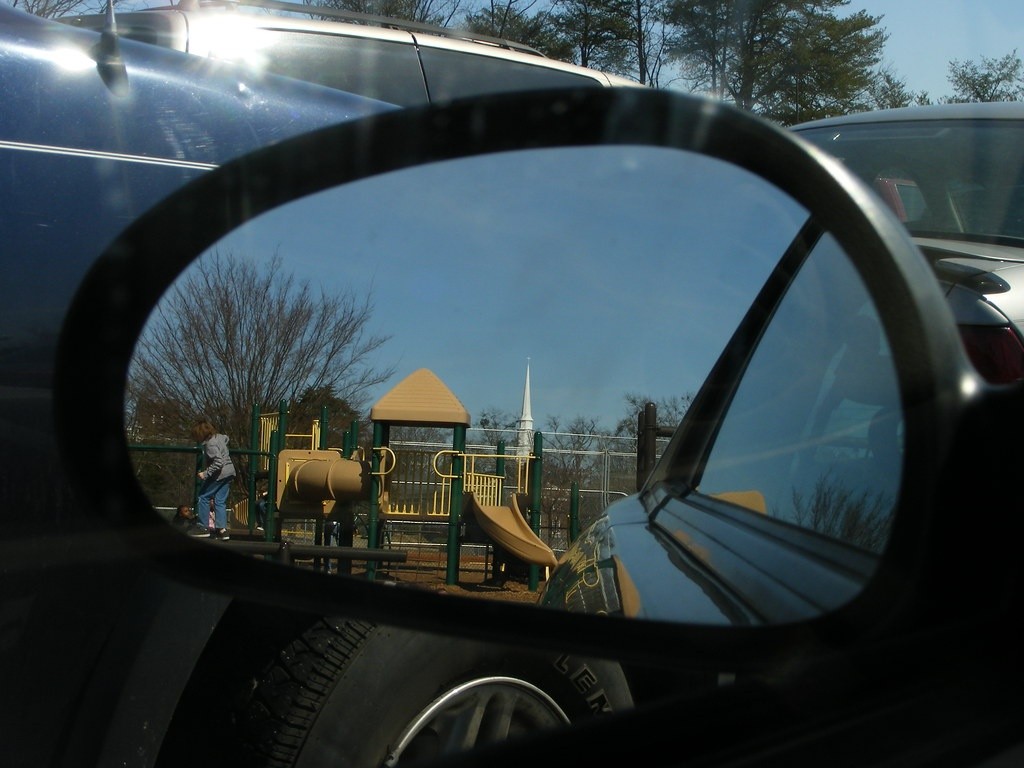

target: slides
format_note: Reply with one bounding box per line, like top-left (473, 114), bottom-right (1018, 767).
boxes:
top-left (473, 491), bottom-right (558, 565)
top-left (230, 491), bottom-right (267, 530)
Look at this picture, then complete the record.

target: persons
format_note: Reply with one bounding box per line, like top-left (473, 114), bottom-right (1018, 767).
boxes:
top-left (171, 505), bottom-right (199, 535)
top-left (191, 423), bottom-right (237, 540)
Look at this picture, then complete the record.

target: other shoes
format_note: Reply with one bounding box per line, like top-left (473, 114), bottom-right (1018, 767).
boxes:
top-left (256, 524), bottom-right (265, 530)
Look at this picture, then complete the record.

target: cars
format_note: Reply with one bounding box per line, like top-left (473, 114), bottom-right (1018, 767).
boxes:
top-left (0, 2), bottom-right (1021, 768)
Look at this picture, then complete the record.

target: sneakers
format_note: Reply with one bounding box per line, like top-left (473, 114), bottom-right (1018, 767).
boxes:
top-left (211, 528), bottom-right (229, 540)
top-left (187, 526), bottom-right (210, 538)
